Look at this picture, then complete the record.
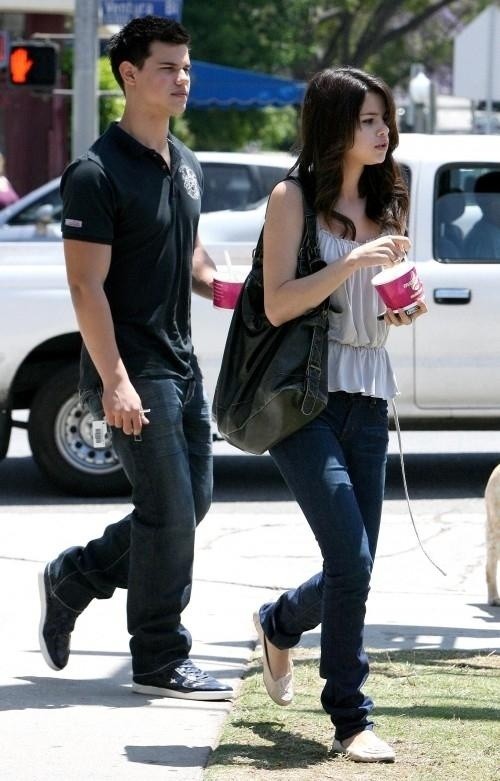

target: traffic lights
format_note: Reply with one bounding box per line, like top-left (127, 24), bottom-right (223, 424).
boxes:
top-left (7, 43), bottom-right (60, 93)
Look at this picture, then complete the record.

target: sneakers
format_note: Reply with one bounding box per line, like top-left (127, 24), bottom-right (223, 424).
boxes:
top-left (332, 738), bottom-right (395, 761)
top-left (133, 657), bottom-right (233, 699)
top-left (253, 609), bottom-right (293, 706)
top-left (38, 564), bottom-right (79, 670)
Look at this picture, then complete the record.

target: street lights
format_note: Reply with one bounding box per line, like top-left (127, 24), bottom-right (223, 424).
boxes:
top-left (410, 71), bottom-right (433, 133)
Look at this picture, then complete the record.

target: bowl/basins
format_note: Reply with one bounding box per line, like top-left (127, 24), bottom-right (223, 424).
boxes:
top-left (369, 262), bottom-right (424, 313)
top-left (214, 265), bottom-right (253, 312)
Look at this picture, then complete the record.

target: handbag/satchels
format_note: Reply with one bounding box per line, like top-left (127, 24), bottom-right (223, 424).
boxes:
top-left (212, 259), bottom-right (328, 454)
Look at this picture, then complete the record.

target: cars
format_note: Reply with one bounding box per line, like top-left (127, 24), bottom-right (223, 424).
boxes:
top-left (2, 151), bottom-right (302, 246)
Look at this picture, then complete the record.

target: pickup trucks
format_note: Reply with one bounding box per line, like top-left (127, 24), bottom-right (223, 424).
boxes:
top-left (1, 129), bottom-right (500, 499)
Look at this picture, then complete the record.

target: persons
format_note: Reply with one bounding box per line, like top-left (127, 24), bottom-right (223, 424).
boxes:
top-left (39, 15), bottom-right (239, 703)
top-left (465, 172), bottom-right (500, 263)
top-left (244, 69), bottom-right (425, 768)
